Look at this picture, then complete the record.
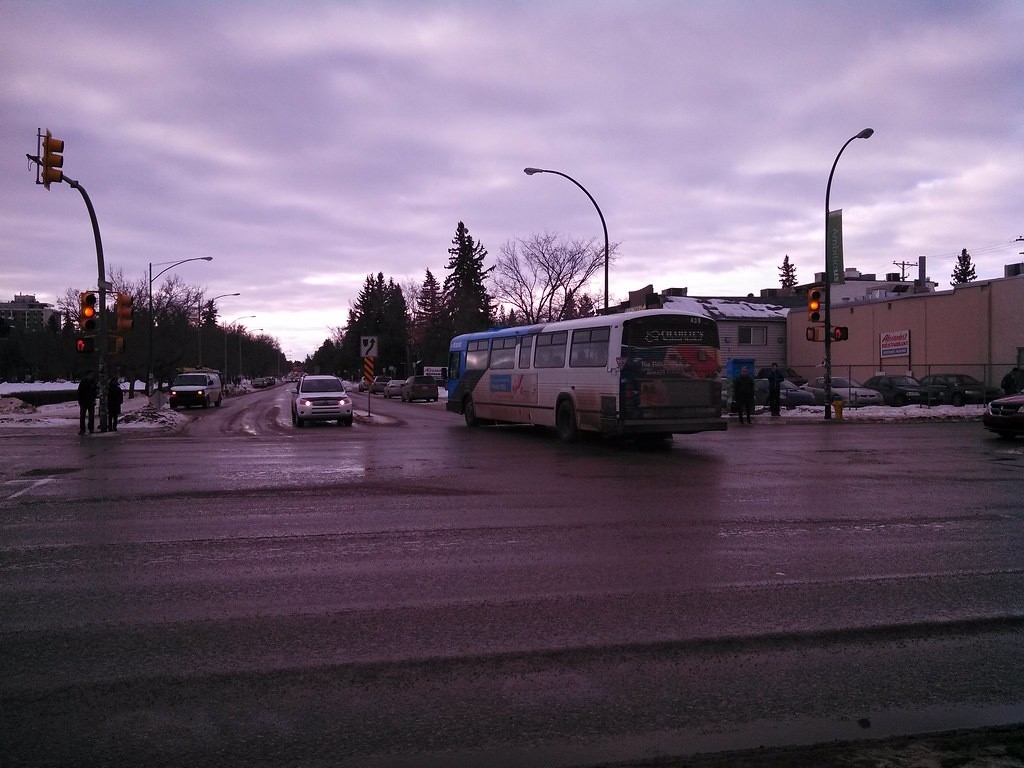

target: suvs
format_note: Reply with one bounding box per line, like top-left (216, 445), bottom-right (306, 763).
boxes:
top-left (400, 375), bottom-right (438, 403)
top-left (290, 372), bottom-right (353, 428)
top-left (357, 375), bottom-right (375, 392)
top-left (368, 375), bottom-right (393, 395)
top-left (756, 367), bottom-right (809, 387)
top-left (169, 370), bottom-right (224, 410)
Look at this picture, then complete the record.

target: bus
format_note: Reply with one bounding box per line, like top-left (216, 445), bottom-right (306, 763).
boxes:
top-left (446, 308), bottom-right (728, 445)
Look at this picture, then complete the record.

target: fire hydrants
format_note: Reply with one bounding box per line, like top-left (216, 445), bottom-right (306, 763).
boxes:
top-left (832, 395), bottom-right (844, 420)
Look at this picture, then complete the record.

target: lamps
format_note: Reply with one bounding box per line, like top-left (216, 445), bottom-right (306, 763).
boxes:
top-left (725, 337), bottom-right (730, 342)
top-left (779, 337), bottom-right (784, 344)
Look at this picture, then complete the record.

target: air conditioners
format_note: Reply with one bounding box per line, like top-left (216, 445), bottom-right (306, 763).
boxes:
top-left (887, 273), bottom-right (899, 281)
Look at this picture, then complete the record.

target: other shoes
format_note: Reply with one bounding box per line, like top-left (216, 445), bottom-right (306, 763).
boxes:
top-left (747, 419), bottom-right (752, 424)
top-left (90, 430), bottom-right (95, 434)
top-left (78, 429), bottom-right (86, 434)
top-left (740, 419), bottom-right (744, 424)
top-left (776, 411), bottom-right (781, 416)
top-left (772, 411), bottom-right (776, 416)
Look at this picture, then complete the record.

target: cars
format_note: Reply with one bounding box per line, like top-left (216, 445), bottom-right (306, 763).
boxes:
top-left (251, 376), bottom-right (276, 389)
top-left (918, 373), bottom-right (989, 407)
top-left (383, 379), bottom-right (406, 399)
top-left (798, 376), bottom-right (884, 408)
top-left (860, 374), bottom-right (933, 407)
top-left (753, 378), bottom-right (817, 408)
top-left (983, 388), bottom-right (1024, 440)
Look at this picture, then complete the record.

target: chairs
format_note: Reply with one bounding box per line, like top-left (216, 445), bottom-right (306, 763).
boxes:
top-left (535, 357), bottom-right (595, 367)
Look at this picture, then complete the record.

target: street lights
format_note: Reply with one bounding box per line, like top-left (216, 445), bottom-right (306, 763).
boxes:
top-left (146, 256), bottom-right (214, 395)
top-left (198, 293), bottom-right (240, 369)
top-left (823, 128), bottom-right (875, 420)
top-left (224, 315), bottom-right (256, 396)
top-left (239, 328), bottom-right (264, 381)
top-left (523, 167), bottom-right (608, 308)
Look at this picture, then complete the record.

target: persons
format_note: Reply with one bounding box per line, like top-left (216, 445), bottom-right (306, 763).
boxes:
top-left (733, 367), bottom-right (755, 424)
top-left (1004, 368), bottom-right (1020, 395)
top-left (75, 370), bottom-right (98, 435)
top-left (768, 362), bottom-right (784, 416)
top-left (107, 377), bottom-right (123, 432)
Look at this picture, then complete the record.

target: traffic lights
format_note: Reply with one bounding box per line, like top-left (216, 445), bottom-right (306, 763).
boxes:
top-left (834, 326), bottom-right (849, 341)
top-left (115, 336), bottom-right (126, 353)
top-left (79, 291), bottom-right (97, 331)
top-left (41, 128), bottom-right (64, 191)
top-left (116, 293), bottom-right (135, 329)
top-left (807, 288), bottom-right (822, 322)
top-left (806, 327), bottom-right (819, 342)
top-left (77, 337), bottom-right (93, 354)
